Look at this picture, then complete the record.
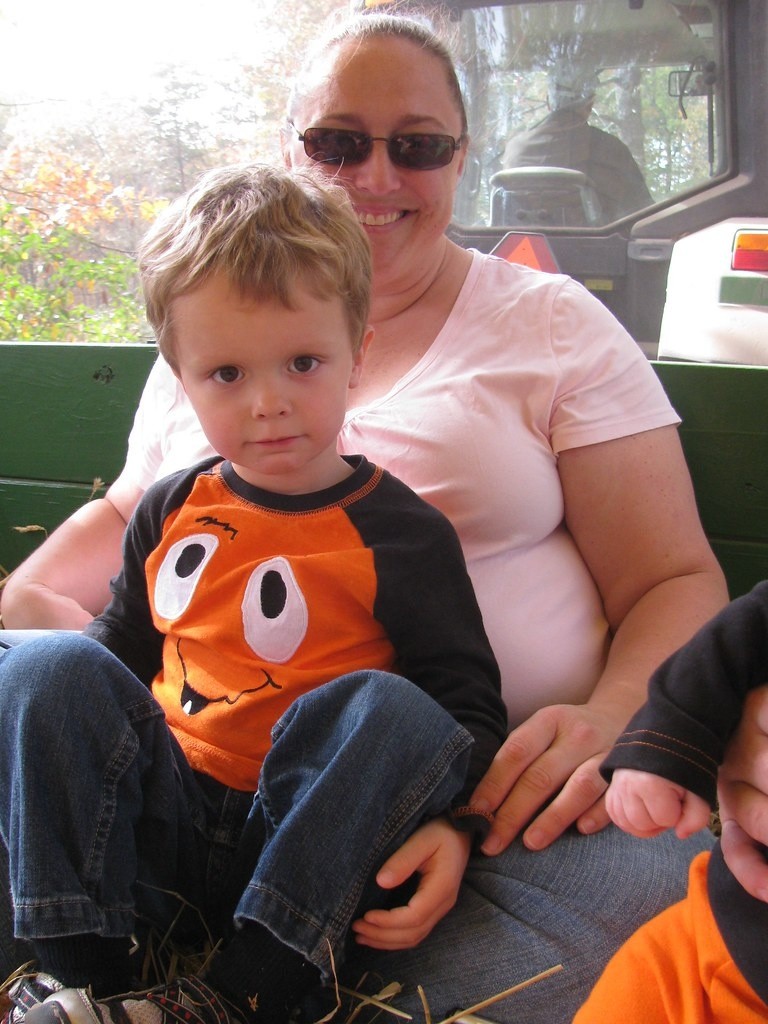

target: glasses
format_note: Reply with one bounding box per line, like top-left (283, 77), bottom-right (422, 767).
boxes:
top-left (289, 120), bottom-right (464, 170)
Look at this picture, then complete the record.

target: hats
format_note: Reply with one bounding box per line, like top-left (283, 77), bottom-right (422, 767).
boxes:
top-left (548, 49), bottom-right (600, 100)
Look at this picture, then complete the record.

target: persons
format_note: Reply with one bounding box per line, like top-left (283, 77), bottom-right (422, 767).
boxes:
top-left (500, 57), bottom-right (655, 226)
top-left (0, 15), bottom-right (732, 1024)
top-left (571, 580), bottom-right (768, 1023)
top-left (718, 684), bottom-right (768, 903)
top-left (0, 160), bottom-right (512, 1023)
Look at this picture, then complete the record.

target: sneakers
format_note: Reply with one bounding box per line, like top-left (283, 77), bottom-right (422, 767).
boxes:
top-left (23, 978), bottom-right (248, 1023)
top-left (7, 967), bottom-right (65, 1024)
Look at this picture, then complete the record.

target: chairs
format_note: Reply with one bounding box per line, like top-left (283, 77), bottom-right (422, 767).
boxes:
top-left (489, 166), bottom-right (607, 232)
